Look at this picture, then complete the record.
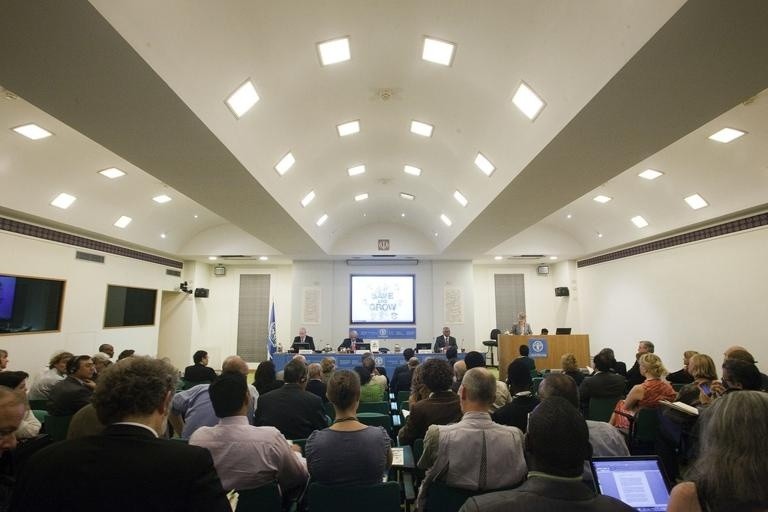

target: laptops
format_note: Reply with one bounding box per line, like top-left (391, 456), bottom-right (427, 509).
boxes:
top-left (585, 452), bottom-right (671, 511)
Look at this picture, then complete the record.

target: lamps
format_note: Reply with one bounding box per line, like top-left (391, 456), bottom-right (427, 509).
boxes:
top-left (536, 264), bottom-right (549, 274)
top-left (212, 264), bottom-right (227, 277)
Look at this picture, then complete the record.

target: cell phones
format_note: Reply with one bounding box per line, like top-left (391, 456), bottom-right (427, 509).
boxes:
top-left (699, 382), bottom-right (713, 398)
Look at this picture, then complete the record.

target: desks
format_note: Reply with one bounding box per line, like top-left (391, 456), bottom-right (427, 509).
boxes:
top-left (269, 350), bottom-right (487, 385)
top-left (495, 332), bottom-right (591, 380)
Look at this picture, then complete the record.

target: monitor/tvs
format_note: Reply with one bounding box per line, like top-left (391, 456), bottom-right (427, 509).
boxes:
top-left (355, 343), bottom-right (370, 350)
top-left (0, 271), bottom-right (68, 338)
top-left (102, 282), bottom-right (159, 329)
top-left (556, 328), bottom-right (572, 334)
top-left (416, 343), bottom-right (431, 352)
top-left (293, 343), bottom-right (310, 353)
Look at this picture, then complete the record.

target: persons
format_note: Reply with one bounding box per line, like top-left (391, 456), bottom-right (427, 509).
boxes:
top-left (289, 327), bottom-right (315, 351)
top-left (505, 313), bottom-right (533, 336)
top-left (2, 341), bottom-right (768, 512)
top-left (434, 327), bottom-right (458, 353)
top-left (337, 329), bottom-right (365, 352)
top-left (539, 329), bottom-right (549, 335)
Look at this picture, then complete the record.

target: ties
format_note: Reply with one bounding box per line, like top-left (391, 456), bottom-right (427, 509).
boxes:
top-left (446, 337), bottom-right (448, 343)
top-left (302, 339), bottom-right (303, 341)
top-left (353, 341), bottom-right (354, 345)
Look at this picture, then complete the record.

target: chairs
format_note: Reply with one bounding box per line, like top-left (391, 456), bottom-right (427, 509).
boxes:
top-left (482, 330), bottom-right (502, 370)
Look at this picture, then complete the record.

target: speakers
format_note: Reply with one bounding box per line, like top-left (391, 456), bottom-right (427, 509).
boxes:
top-left (555, 287), bottom-right (569, 297)
top-left (193, 287), bottom-right (208, 299)
